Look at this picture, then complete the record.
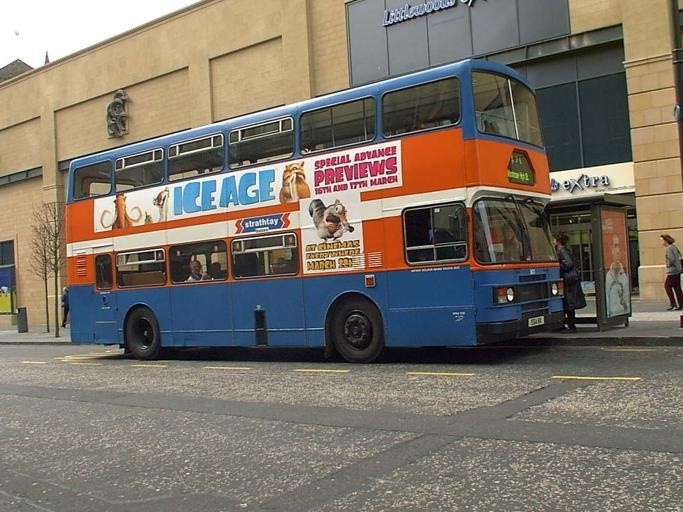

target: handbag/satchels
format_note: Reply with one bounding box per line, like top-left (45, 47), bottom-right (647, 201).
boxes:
top-left (680, 256), bottom-right (683, 274)
top-left (564, 268), bottom-right (579, 286)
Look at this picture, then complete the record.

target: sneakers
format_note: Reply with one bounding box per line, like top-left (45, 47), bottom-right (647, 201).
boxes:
top-left (666, 305), bottom-right (682, 310)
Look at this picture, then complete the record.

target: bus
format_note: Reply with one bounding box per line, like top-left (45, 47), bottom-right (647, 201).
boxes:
top-left (64, 58), bottom-right (565, 363)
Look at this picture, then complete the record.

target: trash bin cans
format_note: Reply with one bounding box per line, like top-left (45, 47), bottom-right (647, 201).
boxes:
top-left (16, 307), bottom-right (27, 333)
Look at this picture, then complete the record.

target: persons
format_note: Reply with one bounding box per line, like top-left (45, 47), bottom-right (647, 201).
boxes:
top-left (551, 232), bottom-right (586, 333)
top-left (659, 233), bottom-right (682, 310)
top-left (60, 286), bottom-right (70, 328)
top-left (556, 230), bottom-right (582, 284)
top-left (605, 232), bottom-right (630, 318)
